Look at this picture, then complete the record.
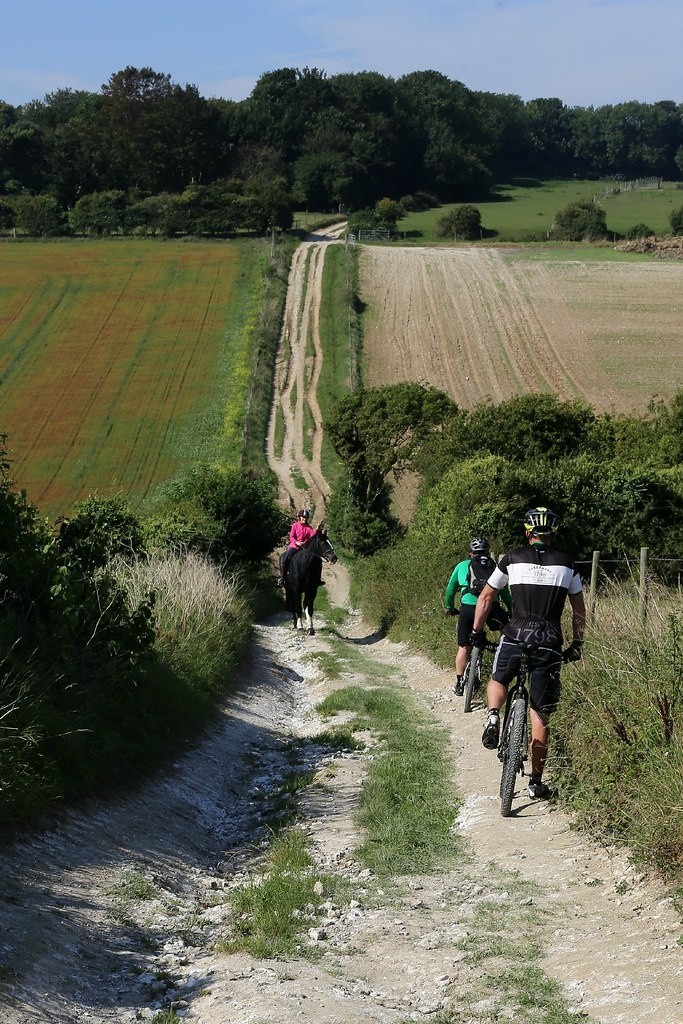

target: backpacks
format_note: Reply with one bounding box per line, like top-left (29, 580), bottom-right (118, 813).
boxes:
top-left (461, 555), bottom-right (502, 599)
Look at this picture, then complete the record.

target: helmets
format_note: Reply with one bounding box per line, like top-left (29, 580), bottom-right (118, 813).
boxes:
top-left (523, 507), bottom-right (558, 535)
top-left (470, 538), bottom-right (493, 554)
top-left (298, 510), bottom-right (309, 520)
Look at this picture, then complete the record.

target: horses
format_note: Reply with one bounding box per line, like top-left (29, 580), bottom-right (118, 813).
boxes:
top-left (283, 525), bottom-right (337, 636)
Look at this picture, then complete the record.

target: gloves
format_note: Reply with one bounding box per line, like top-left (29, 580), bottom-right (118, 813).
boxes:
top-left (469, 631), bottom-right (489, 647)
top-left (446, 607), bottom-right (459, 616)
top-left (563, 640), bottom-right (584, 662)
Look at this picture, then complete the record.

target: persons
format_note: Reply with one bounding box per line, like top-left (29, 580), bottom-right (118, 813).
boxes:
top-left (470, 507), bottom-right (586, 800)
top-left (445, 538), bottom-right (511, 696)
top-left (277, 510), bottom-right (326, 586)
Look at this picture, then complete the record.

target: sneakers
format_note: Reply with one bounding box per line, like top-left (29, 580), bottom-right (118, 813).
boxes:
top-left (482, 715), bottom-right (500, 749)
top-left (527, 777), bottom-right (543, 800)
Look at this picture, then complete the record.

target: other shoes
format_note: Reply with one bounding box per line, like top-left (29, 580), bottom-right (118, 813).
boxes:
top-left (277, 578), bottom-right (284, 586)
top-left (319, 580), bottom-right (325, 585)
top-left (455, 683), bottom-right (464, 695)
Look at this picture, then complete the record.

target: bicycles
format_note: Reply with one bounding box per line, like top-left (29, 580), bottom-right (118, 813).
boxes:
top-left (476, 640), bottom-right (578, 817)
top-left (446, 609), bottom-right (504, 713)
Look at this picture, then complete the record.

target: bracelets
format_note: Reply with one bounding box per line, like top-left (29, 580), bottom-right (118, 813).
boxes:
top-left (473, 628), bottom-right (483, 633)
